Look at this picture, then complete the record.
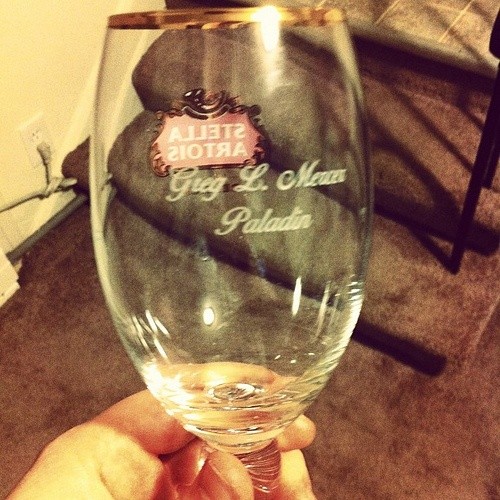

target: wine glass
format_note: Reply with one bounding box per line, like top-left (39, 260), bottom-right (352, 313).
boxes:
top-left (89, 7), bottom-right (375, 499)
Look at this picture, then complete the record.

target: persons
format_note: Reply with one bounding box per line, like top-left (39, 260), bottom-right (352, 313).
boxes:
top-left (2, 360), bottom-right (320, 500)
